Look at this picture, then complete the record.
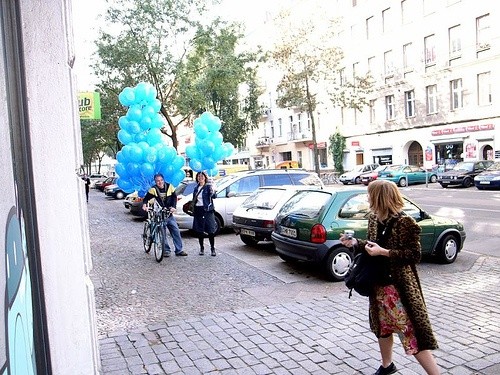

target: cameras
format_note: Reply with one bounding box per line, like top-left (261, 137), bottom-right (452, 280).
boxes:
top-left (343, 230), bottom-right (355, 241)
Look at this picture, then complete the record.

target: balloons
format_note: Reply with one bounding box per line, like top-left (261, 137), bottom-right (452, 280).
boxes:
top-left (185, 112), bottom-right (233, 177)
top-left (114, 82), bottom-right (186, 198)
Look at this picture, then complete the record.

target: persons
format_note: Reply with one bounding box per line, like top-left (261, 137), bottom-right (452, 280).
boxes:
top-left (340, 180), bottom-right (440, 375)
top-left (84, 174), bottom-right (90, 202)
top-left (143, 174), bottom-right (188, 257)
top-left (191, 171), bottom-right (218, 256)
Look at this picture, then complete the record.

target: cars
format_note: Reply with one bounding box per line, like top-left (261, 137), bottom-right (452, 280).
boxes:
top-left (437, 158), bottom-right (495, 188)
top-left (88, 163), bottom-right (250, 219)
top-left (271, 183), bottom-right (467, 282)
top-left (339, 163), bottom-right (381, 185)
top-left (231, 184), bottom-right (313, 248)
top-left (360, 158), bottom-right (463, 187)
top-left (473, 160), bottom-right (500, 191)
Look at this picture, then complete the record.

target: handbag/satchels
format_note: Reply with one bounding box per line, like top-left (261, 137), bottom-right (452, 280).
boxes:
top-left (183, 200), bottom-right (196, 216)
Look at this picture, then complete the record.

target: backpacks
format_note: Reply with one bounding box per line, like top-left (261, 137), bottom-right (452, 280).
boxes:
top-left (344, 252), bottom-right (376, 298)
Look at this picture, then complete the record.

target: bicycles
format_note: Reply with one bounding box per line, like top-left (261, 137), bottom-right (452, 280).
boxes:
top-left (140, 206), bottom-right (173, 263)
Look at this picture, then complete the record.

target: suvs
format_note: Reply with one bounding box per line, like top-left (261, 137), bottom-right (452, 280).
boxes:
top-left (173, 168), bottom-right (324, 237)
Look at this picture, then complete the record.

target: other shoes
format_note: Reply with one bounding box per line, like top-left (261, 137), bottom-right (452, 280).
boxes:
top-left (176, 251), bottom-right (187, 256)
top-left (163, 250), bottom-right (170, 256)
top-left (211, 248), bottom-right (216, 256)
top-left (371, 362), bottom-right (398, 375)
top-left (199, 246), bottom-right (204, 255)
